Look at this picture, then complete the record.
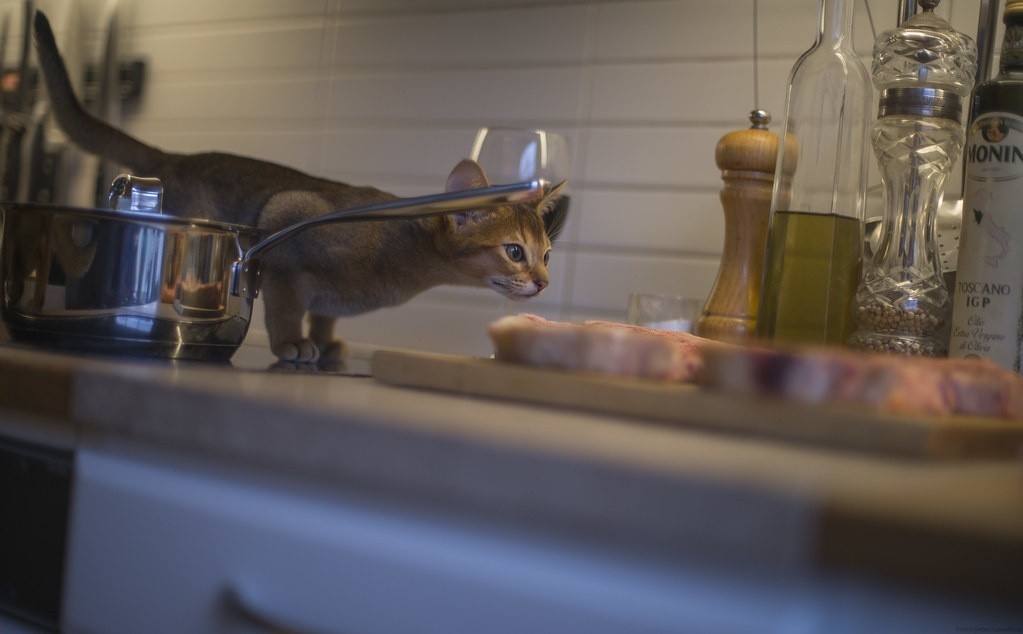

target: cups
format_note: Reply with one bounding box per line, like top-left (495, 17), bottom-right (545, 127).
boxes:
top-left (627, 293), bottom-right (706, 334)
top-left (470, 126), bottom-right (574, 247)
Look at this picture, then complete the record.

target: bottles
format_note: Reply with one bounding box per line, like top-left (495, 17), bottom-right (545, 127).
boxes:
top-left (691, 0), bottom-right (1023, 374)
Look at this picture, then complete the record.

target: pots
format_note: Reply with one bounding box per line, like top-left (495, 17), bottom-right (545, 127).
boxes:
top-left (0, 173), bottom-right (548, 362)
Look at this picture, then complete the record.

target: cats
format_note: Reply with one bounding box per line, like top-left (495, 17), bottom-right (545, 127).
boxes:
top-left (34, 8), bottom-right (570, 361)
